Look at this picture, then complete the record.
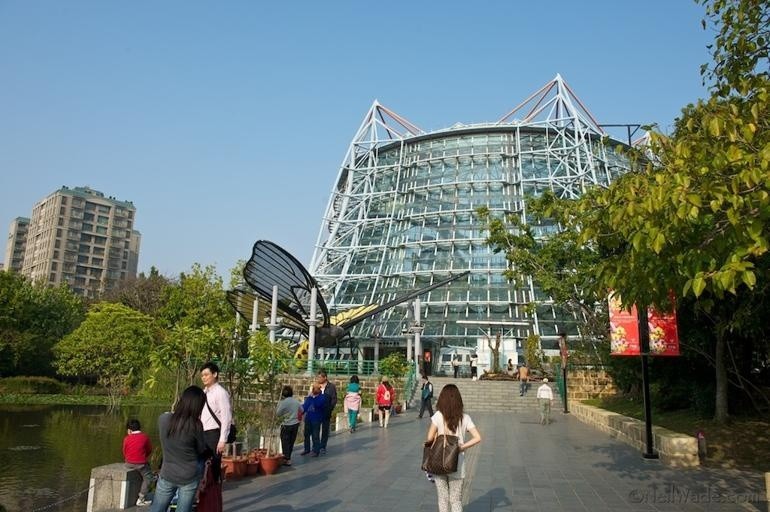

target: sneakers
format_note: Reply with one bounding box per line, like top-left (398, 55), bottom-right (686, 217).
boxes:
top-left (136, 497), bottom-right (153, 508)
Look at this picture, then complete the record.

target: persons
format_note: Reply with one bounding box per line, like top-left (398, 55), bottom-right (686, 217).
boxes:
top-left (423, 384), bottom-right (482, 512)
top-left (314, 370), bottom-right (337, 454)
top-left (537, 378), bottom-right (554, 425)
top-left (276, 385), bottom-right (305, 465)
top-left (517, 361), bottom-right (531, 397)
top-left (469, 354), bottom-right (480, 381)
top-left (452, 354), bottom-right (462, 379)
top-left (300, 384), bottom-right (326, 458)
top-left (193, 361), bottom-right (233, 512)
top-left (147, 385), bottom-right (206, 512)
top-left (343, 375), bottom-right (362, 433)
top-left (507, 358), bottom-right (514, 377)
top-left (122, 418), bottom-right (154, 506)
top-left (416, 375), bottom-right (434, 419)
top-left (376, 376), bottom-right (395, 429)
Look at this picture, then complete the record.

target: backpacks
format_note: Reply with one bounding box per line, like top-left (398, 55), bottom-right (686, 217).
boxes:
top-left (384, 391), bottom-right (391, 402)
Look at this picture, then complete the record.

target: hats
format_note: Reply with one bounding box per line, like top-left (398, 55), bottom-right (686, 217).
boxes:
top-left (542, 378), bottom-right (548, 383)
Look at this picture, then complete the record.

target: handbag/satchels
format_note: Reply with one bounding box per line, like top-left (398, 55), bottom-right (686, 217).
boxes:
top-left (225, 425), bottom-right (238, 445)
top-left (421, 433), bottom-right (461, 476)
